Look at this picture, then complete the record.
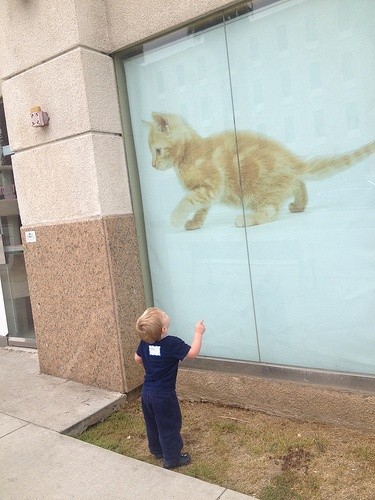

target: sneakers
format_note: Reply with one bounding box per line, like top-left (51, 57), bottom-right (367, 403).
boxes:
top-left (163, 452), bottom-right (191, 469)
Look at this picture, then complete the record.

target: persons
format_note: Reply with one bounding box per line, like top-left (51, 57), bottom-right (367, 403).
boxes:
top-left (134, 307), bottom-right (205, 469)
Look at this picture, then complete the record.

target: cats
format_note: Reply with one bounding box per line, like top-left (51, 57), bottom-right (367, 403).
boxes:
top-left (142, 112), bottom-right (374, 230)
